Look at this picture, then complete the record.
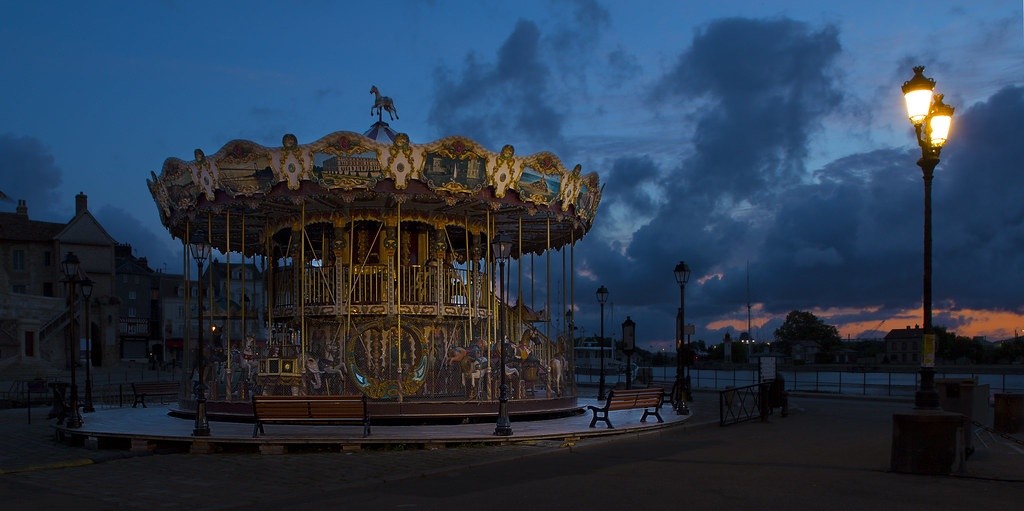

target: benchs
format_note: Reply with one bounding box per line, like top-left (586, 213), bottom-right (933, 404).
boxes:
top-left (647, 380), bottom-right (676, 404)
top-left (132, 382), bottom-right (181, 408)
top-left (252, 394), bottom-right (371, 438)
top-left (588, 388), bottom-right (665, 429)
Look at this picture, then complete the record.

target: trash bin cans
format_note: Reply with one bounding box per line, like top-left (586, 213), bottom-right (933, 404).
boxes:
top-left (936, 378), bottom-right (973, 412)
top-left (48, 380), bottom-right (77, 414)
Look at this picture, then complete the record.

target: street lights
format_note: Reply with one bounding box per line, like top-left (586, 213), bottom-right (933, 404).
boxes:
top-left (565, 309), bottom-right (573, 337)
top-left (892, 65), bottom-right (966, 476)
top-left (187, 225), bottom-right (211, 437)
top-left (79, 275), bottom-right (95, 414)
top-left (491, 229), bottom-right (515, 437)
top-left (672, 261), bottom-right (691, 416)
top-left (595, 284), bottom-right (609, 400)
top-left (61, 250), bottom-right (85, 428)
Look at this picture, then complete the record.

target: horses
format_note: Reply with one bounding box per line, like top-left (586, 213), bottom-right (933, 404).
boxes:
top-left (192, 324), bottom-right (571, 398)
top-left (369, 85), bottom-right (399, 120)
